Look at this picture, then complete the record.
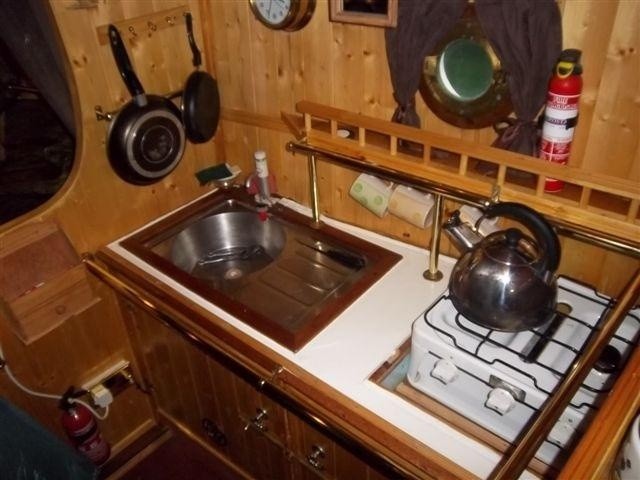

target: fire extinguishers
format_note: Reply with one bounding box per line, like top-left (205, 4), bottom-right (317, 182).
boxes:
top-left (57, 385), bottom-right (111, 466)
top-left (537, 49), bottom-right (584, 193)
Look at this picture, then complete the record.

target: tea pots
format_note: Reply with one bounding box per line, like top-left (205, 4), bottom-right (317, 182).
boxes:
top-left (440, 200), bottom-right (561, 334)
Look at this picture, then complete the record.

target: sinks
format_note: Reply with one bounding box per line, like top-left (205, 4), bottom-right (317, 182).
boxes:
top-left (142, 195), bottom-right (288, 282)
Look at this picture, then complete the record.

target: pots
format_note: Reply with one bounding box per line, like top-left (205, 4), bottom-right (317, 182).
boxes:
top-left (182, 12), bottom-right (221, 145)
top-left (105, 24), bottom-right (186, 186)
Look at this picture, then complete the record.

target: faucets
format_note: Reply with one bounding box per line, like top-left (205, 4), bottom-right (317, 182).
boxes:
top-left (245, 150), bottom-right (274, 200)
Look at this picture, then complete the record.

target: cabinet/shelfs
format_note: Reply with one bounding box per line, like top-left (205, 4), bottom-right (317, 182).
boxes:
top-left (117, 286), bottom-right (381, 480)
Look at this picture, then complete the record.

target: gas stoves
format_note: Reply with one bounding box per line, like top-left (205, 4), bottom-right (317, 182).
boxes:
top-left (408, 274), bottom-right (640, 466)
